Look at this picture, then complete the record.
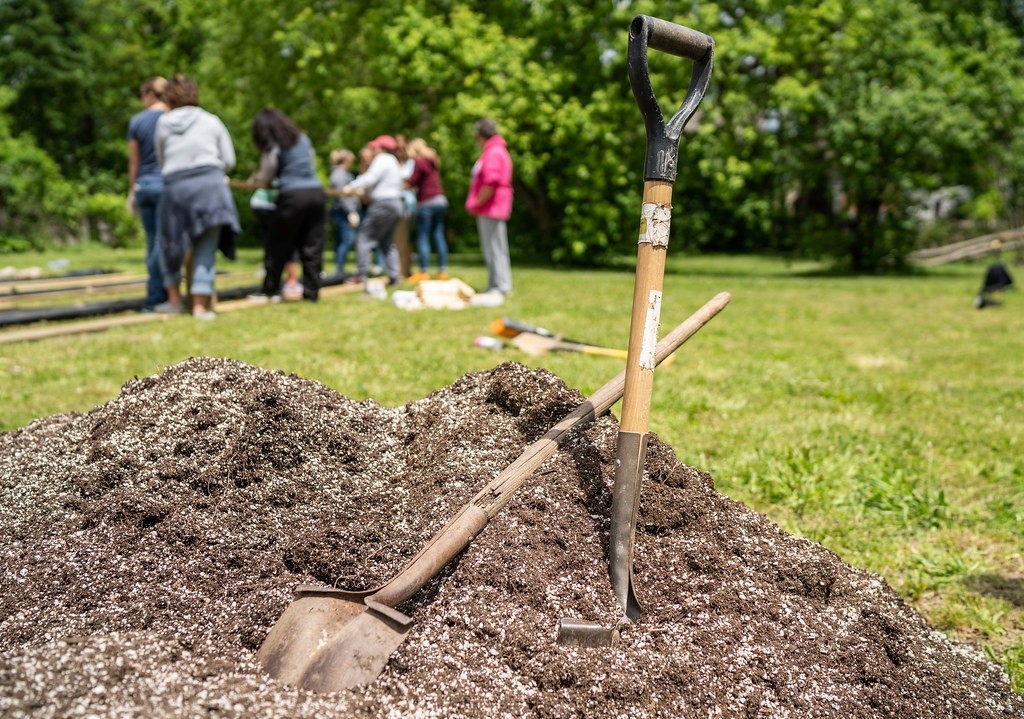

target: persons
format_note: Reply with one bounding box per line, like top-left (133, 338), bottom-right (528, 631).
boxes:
top-left (330, 134), bottom-right (415, 278)
top-left (126, 77), bottom-right (189, 311)
top-left (343, 135), bottom-right (402, 287)
top-left (249, 188), bottom-right (304, 294)
top-left (228, 106), bottom-right (325, 301)
top-left (152, 74), bottom-right (245, 317)
top-left (400, 138), bottom-right (449, 281)
top-left (465, 120), bottom-right (514, 306)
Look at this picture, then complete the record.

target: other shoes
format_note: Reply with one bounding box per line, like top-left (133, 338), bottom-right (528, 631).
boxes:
top-left (483, 285), bottom-right (508, 296)
top-left (436, 273), bottom-right (449, 280)
top-left (154, 300), bottom-right (186, 314)
top-left (193, 309), bottom-right (218, 322)
top-left (406, 272), bottom-right (430, 283)
top-left (247, 292), bottom-right (282, 302)
top-left (345, 276), bottom-right (357, 284)
top-left (283, 281), bottom-right (304, 301)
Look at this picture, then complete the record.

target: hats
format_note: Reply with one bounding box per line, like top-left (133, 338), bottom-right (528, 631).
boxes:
top-left (368, 135), bottom-right (397, 150)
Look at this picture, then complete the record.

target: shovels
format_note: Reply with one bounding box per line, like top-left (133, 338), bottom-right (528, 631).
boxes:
top-left (556, 14), bottom-right (718, 651)
top-left (254, 288), bottom-right (735, 694)
